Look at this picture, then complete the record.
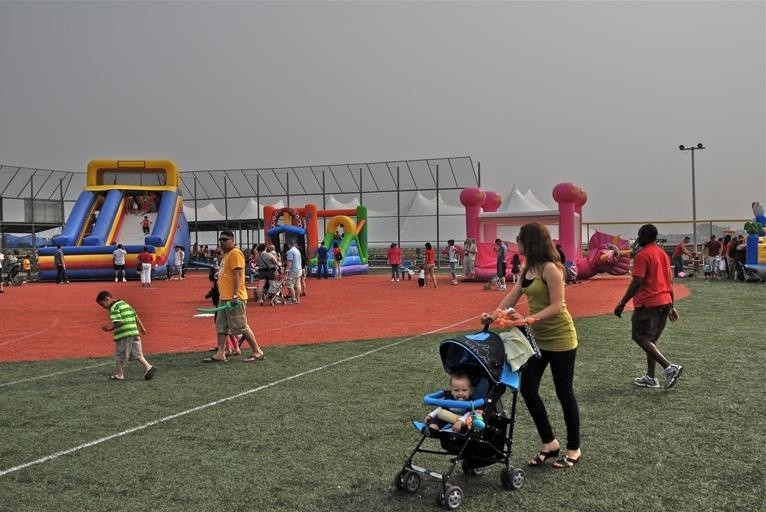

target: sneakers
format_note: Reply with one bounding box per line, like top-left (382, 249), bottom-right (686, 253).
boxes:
top-left (390, 278), bottom-right (400, 282)
top-left (633, 364), bottom-right (683, 389)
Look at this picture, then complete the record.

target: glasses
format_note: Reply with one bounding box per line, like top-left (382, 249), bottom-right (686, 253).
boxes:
top-left (219, 238), bottom-right (229, 241)
top-left (517, 236), bottom-right (521, 243)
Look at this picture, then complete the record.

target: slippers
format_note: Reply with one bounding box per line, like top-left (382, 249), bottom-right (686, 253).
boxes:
top-left (243, 356), bottom-right (266, 362)
top-left (284, 295), bottom-right (291, 298)
top-left (203, 356), bottom-right (227, 362)
top-left (110, 375), bottom-right (123, 380)
top-left (144, 366), bottom-right (156, 380)
top-left (528, 450), bottom-right (580, 468)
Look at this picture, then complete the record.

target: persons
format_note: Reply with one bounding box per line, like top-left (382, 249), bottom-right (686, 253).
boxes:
top-left (480, 221), bottom-right (581, 469)
top-left (672, 234), bottom-right (746, 281)
top-left (614, 224), bottom-right (683, 389)
top-left (556, 244), bottom-right (565, 265)
top-left (96, 291), bottom-right (156, 380)
top-left (112, 242), bottom-right (228, 287)
top-left (203, 232), bottom-right (264, 363)
top-left (124, 191), bottom-right (160, 235)
top-left (209, 246), bottom-right (246, 351)
top-left (248, 222), bottom-right (345, 307)
top-left (205, 269), bottom-right (242, 356)
top-left (423, 371), bottom-right (474, 432)
top-left (387, 238), bottom-right (521, 291)
top-left (90, 208), bottom-right (100, 229)
top-left (0, 245), bottom-right (70, 293)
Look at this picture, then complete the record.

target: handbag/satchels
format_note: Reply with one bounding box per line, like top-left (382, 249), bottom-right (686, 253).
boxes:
top-left (136, 263), bottom-right (142, 271)
top-left (272, 271), bottom-right (279, 279)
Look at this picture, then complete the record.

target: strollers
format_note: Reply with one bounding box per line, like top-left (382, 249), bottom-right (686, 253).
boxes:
top-left (260, 270), bottom-right (290, 307)
top-left (387, 312), bottom-right (540, 506)
top-left (1, 259), bottom-right (22, 288)
top-left (564, 264), bottom-right (578, 284)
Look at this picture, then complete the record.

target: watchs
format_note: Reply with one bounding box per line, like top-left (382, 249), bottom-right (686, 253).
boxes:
top-left (617, 300), bottom-right (625, 308)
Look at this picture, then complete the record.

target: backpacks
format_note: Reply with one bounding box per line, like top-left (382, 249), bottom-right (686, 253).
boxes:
top-left (336, 252), bottom-right (342, 261)
